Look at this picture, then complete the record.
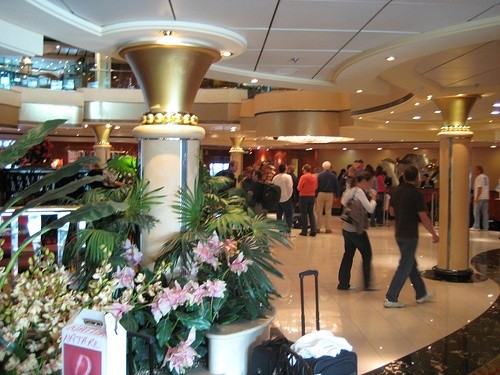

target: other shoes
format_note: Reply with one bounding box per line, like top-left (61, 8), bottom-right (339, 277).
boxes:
top-left (299, 231), bottom-right (307, 236)
top-left (317, 229), bottom-right (320, 233)
top-left (481, 228), bottom-right (488, 231)
top-left (368, 288), bottom-right (378, 290)
top-left (469, 227), bottom-right (480, 230)
top-left (325, 230), bottom-right (332, 233)
top-left (349, 286), bottom-right (355, 290)
top-left (309, 232), bottom-right (316, 236)
top-left (376, 221), bottom-right (383, 224)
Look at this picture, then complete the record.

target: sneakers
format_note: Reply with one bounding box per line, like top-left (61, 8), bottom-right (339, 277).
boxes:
top-left (383, 298), bottom-right (405, 308)
top-left (417, 291), bottom-right (433, 303)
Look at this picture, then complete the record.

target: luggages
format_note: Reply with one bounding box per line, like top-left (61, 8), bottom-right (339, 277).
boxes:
top-left (282, 269), bottom-right (357, 375)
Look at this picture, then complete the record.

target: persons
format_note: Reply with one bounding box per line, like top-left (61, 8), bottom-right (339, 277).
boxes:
top-left (286, 165), bottom-right (301, 229)
top-left (383, 166), bottom-right (440, 308)
top-left (469, 165), bottom-right (489, 231)
top-left (296, 164), bottom-right (318, 236)
top-left (421, 174), bottom-right (435, 188)
top-left (337, 172), bottom-right (377, 291)
top-left (215, 160), bottom-right (238, 193)
top-left (244, 170), bottom-right (265, 204)
top-left (272, 164), bottom-right (293, 233)
top-left (313, 160), bottom-right (337, 234)
top-left (337, 159), bottom-right (389, 227)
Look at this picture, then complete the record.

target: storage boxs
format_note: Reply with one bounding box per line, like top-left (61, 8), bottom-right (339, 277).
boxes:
top-left (60, 308), bottom-right (127, 375)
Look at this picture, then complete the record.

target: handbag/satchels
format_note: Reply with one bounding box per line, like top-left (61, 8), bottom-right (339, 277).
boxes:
top-left (340, 188), bottom-right (369, 230)
top-left (250, 338), bottom-right (296, 375)
